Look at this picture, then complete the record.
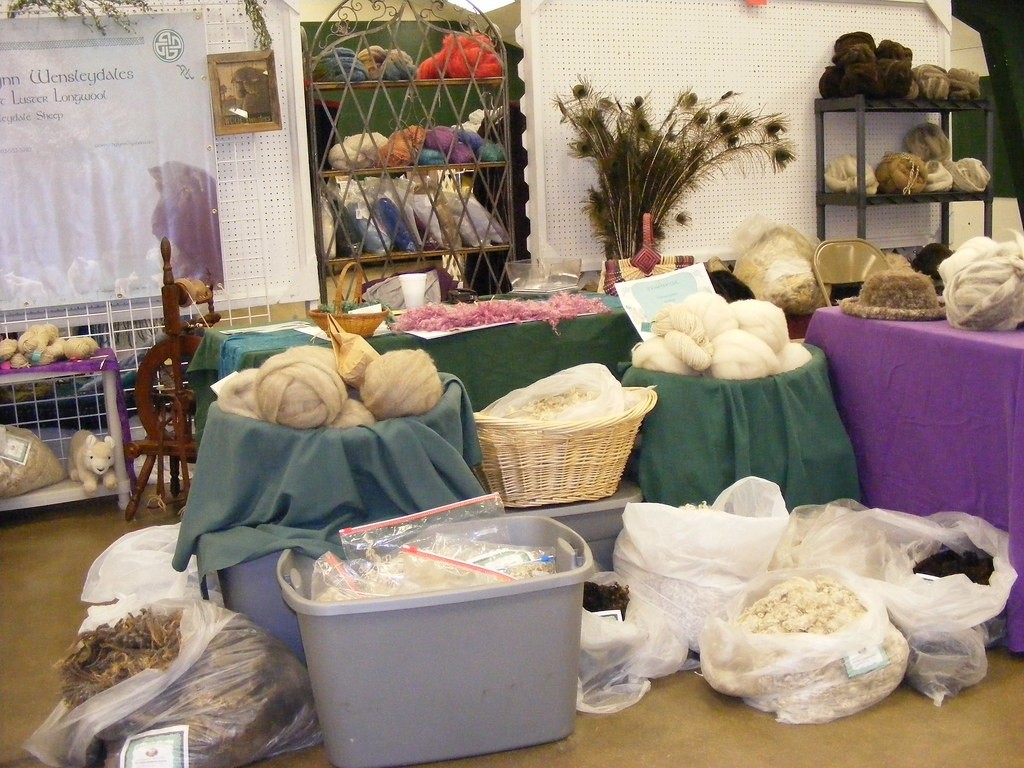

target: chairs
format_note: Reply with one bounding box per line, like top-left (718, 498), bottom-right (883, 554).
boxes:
top-left (811, 238), bottom-right (893, 307)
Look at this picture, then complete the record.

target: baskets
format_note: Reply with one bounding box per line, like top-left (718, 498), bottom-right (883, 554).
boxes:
top-left (473, 385), bottom-right (659, 510)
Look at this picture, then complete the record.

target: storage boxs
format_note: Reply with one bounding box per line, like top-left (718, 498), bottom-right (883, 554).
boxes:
top-left (472, 386), bottom-right (659, 509)
top-left (275, 513), bottom-right (595, 768)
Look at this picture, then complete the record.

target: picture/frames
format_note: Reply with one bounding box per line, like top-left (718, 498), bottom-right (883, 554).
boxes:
top-left (207, 49), bottom-right (283, 136)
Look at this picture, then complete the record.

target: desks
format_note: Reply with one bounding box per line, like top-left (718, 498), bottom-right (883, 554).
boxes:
top-left (803, 294), bottom-right (1024, 656)
top-left (185, 290), bottom-right (644, 457)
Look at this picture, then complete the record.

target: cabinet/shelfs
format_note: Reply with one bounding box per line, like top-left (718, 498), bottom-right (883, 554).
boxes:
top-left (307, 0), bottom-right (517, 307)
top-left (0, 347), bottom-right (132, 512)
top-left (813, 92), bottom-right (995, 250)
